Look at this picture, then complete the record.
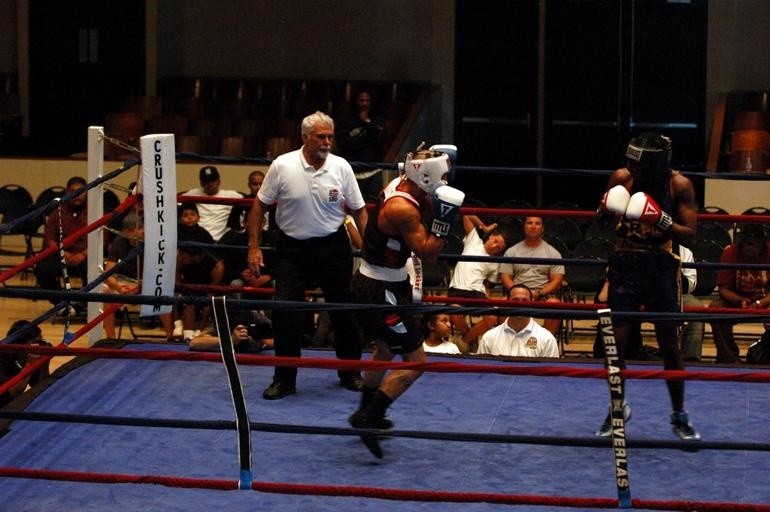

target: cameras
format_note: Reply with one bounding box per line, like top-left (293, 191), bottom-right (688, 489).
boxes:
top-left (34, 339), bottom-right (53, 355)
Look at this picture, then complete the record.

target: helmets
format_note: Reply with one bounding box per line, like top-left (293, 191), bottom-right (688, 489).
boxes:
top-left (405, 150), bottom-right (452, 196)
top-left (625, 133), bottom-right (673, 170)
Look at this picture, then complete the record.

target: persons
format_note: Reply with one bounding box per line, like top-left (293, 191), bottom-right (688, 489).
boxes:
top-left (34, 167), bottom-right (276, 350)
top-left (335, 91), bottom-right (391, 202)
top-left (249, 112), bottom-right (370, 401)
top-left (2, 320), bottom-right (55, 410)
top-left (585, 133), bottom-right (702, 440)
top-left (593, 225), bottom-right (770, 365)
top-left (419, 214), bottom-right (564, 357)
top-left (348, 144), bottom-right (466, 459)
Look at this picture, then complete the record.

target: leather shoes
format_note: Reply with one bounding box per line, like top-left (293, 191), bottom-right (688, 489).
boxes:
top-left (338, 374), bottom-right (366, 392)
top-left (262, 378), bottom-right (296, 399)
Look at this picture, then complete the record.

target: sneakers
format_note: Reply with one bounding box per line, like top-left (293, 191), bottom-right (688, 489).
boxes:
top-left (595, 400), bottom-right (634, 439)
top-left (53, 301), bottom-right (204, 342)
top-left (347, 411), bottom-right (394, 457)
top-left (670, 409), bottom-right (702, 444)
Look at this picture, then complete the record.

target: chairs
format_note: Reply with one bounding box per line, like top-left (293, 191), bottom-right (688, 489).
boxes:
top-left (4, 182), bottom-right (767, 349)
top-left (71, 78), bottom-right (420, 167)
top-left (723, 88), bottom-right (769, 177)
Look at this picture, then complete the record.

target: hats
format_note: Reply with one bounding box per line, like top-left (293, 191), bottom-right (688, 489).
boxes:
top-left (198, 166), bottom-right (221, 184)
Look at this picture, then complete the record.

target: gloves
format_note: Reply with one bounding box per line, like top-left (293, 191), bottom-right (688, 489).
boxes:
top-left (595, 184), bottom-right (629, 223)
top-left (429, 144), bottom-right (458, 167)
top-left (627, 190), bottom-right (673, 232)
top-left (429, 185), bottom-right (465, 238)
